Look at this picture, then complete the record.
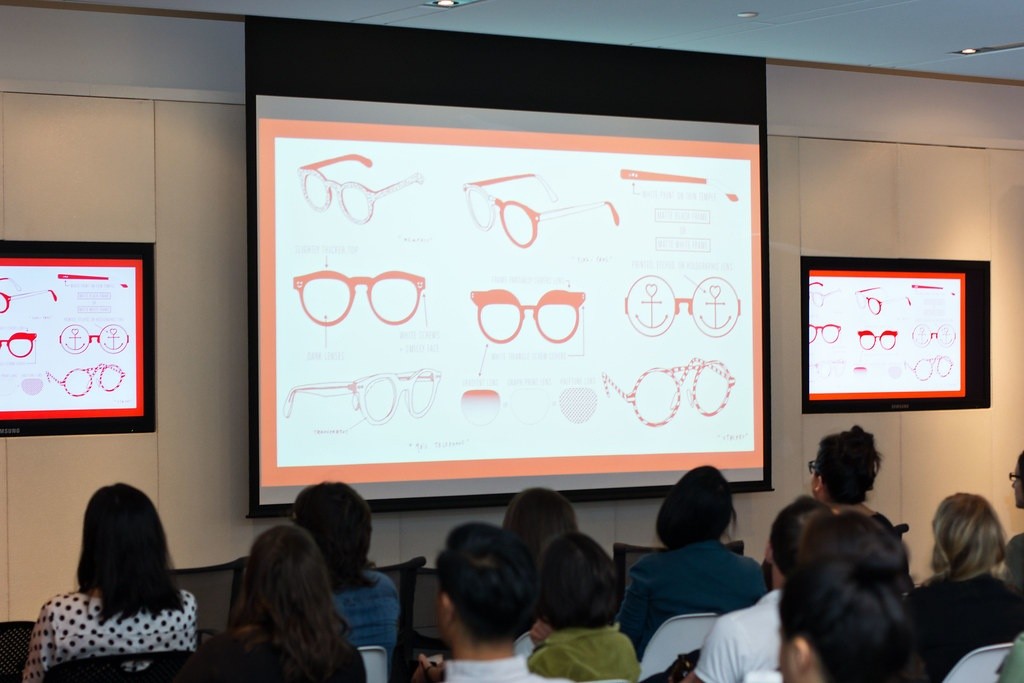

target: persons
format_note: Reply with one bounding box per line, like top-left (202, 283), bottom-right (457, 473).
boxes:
top-left (175, 525), bottom-right (366, 683)
top-left (913, 494), bottom-right (1024, 683)
top-left (761, 426), bottom-right (894, 593)
top-left (617, 466), bottom-right (769, 663)
top-left (291, 481), bottom-right (401, 681)
top-left (796, 509), bottom-right (915, 598)
top-left (779, 552), bottom-right (913, 683)
top-left (410, 522), bottom-right (577, 683)
top-left (1006, 450), bottom-right (1024, 592)
top-left (682, 496), bottom-right (833, 683)
top-left (501, 488), bottom-right (576, 560)
top-left (528, 529), bottom-right (640, 682)
top-left (22, 481), bottom-right (197, 682)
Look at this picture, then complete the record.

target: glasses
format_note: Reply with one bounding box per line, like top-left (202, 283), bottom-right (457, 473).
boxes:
top-left (1008, 471), bottom-right (1024, 482)
top-left (808, 460), bottom-right (828, 485)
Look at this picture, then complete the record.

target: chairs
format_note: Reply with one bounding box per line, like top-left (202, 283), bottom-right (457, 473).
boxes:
top-left (0, 524), bottom-right (1014, 683)
